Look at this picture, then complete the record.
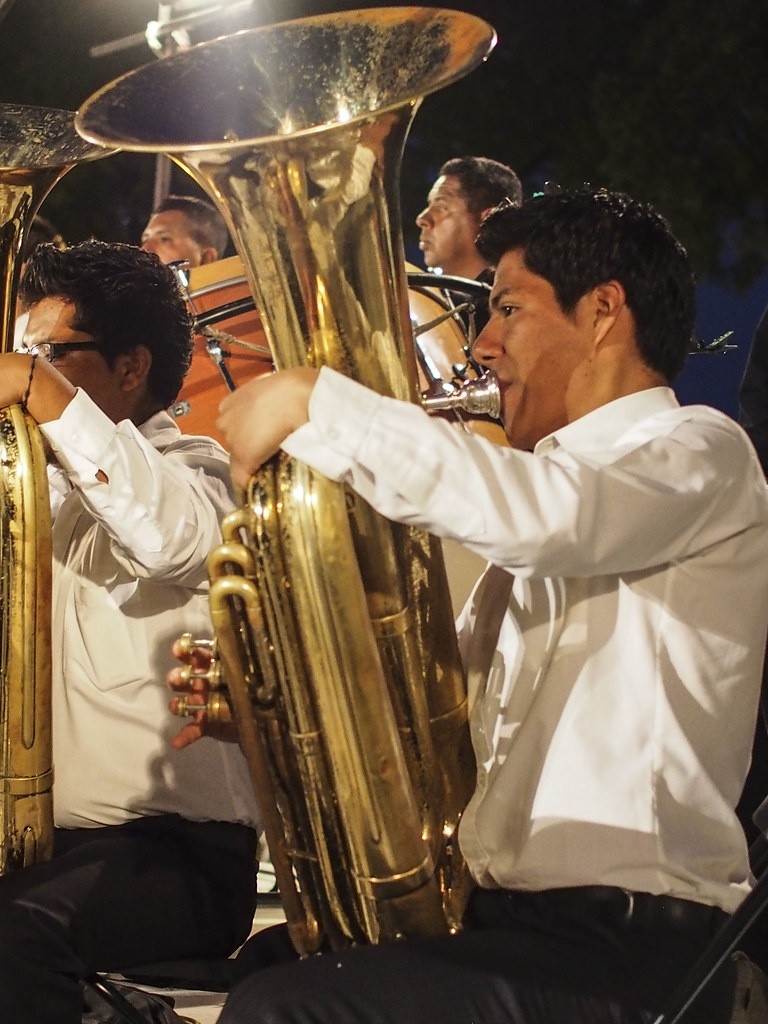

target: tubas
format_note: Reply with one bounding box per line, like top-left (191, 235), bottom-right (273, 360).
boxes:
top-left (0, 99), bottom-right (126, 877)
top-left (71, 0), bottom-right (502, 958)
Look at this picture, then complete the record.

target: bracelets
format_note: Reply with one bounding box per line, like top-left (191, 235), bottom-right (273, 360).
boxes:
top-left (22, 352), bottom-right (37, 412)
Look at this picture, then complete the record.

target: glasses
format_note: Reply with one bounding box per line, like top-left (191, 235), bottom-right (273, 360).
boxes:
top-left (14, 340), bottom-right (97, 363)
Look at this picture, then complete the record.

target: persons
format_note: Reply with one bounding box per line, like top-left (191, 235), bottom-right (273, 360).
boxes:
top-left (0, 157), bottom-right (525, 1024)
top-left (215, 183), bottom-right (767, 1024)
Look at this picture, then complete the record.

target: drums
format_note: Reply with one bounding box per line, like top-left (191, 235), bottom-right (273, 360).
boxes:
top-left (143, 253), bottom-right (513, 454)
